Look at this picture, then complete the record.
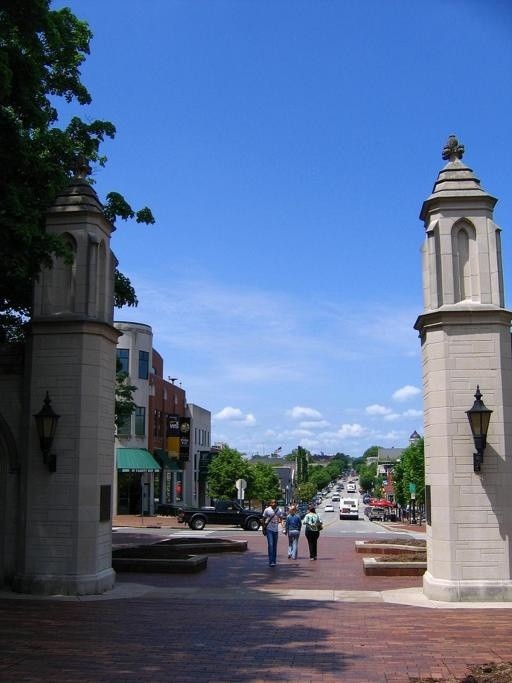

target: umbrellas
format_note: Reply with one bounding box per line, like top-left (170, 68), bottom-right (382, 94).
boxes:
top-left (369, 500), bottom-right (398, 522)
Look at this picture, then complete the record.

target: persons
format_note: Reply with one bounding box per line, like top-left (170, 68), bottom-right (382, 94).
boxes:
top-left (262, 499), bottom-right (285, 566)
top-left (285, 505), bottom-right (301, 560)
top-left (303, 507), bottom-right (322, 561)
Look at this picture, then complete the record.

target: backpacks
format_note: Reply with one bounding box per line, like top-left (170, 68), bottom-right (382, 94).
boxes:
top-left (263, 526), bottom-right (267, 535)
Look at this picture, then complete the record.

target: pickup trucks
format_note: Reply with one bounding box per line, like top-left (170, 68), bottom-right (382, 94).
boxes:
top-left (176, 498), bottom-right (267, 531)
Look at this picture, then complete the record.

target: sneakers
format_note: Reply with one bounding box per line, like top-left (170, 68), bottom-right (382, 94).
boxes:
top-left (309, 556), bottom-right (317, 561)
top-left (288, 554), bottom-right (297, 560)
top-left (269, 563), bottom-right (276, 567)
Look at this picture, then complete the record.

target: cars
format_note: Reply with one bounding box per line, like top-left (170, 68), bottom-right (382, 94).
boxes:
top-left (314, 482), bottom-right (357, 504)
top-left (324, 503), bottom-right (333, 512)
top-left (364, 504), bottom-right (385, 521)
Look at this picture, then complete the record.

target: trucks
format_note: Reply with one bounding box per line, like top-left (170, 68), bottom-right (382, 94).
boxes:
top-left (339, 496), bottom-right (359, 519)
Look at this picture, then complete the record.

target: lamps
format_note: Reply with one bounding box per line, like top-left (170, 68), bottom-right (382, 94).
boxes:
top-left (32, 390), bottom-right (60, 473)
top-left (464, 384), bottom-right (495, 472)
